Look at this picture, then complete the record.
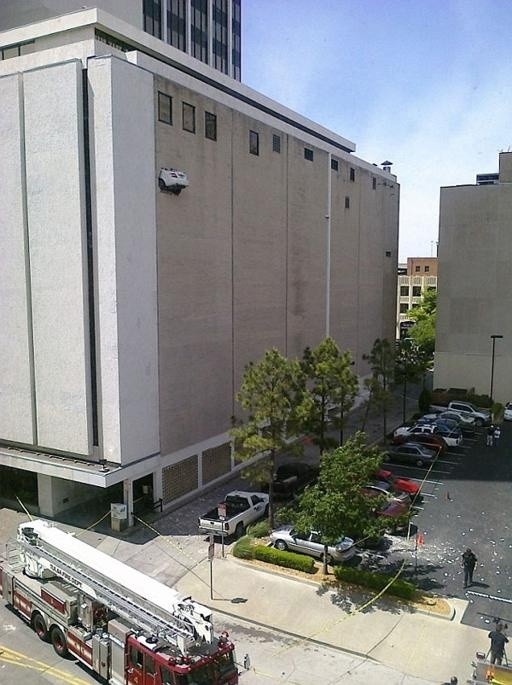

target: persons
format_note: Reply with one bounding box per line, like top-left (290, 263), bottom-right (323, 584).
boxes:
top-left (442, 676), bottom-right (457, 685)
top-left (494, 427), bottom-right (501, 447)
top-left (486, 425), bottom-right (494, 447)
top-left (488, 624), bottom-right (509, 665)
top-left (461, 548), bottom-right (477, 589)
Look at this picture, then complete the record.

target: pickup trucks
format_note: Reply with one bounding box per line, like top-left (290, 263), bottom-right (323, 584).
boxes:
top-left (197, 461), bottom-right (318, 540)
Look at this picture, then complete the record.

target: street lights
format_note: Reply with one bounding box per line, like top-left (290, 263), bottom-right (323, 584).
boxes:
top-left (490, 334), bottom-right (503, 405)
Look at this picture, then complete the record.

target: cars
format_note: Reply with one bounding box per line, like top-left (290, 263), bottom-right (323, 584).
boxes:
top-left (270, 468), bottom-right (421, 563)
top-left (504, 401), bottom-right (512, 421)
top-left (382, 399), bottom-right (492, 467)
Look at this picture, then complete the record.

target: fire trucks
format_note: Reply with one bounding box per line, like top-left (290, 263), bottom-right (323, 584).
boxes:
top-left (0, 520), bottom-right (238, 685)
